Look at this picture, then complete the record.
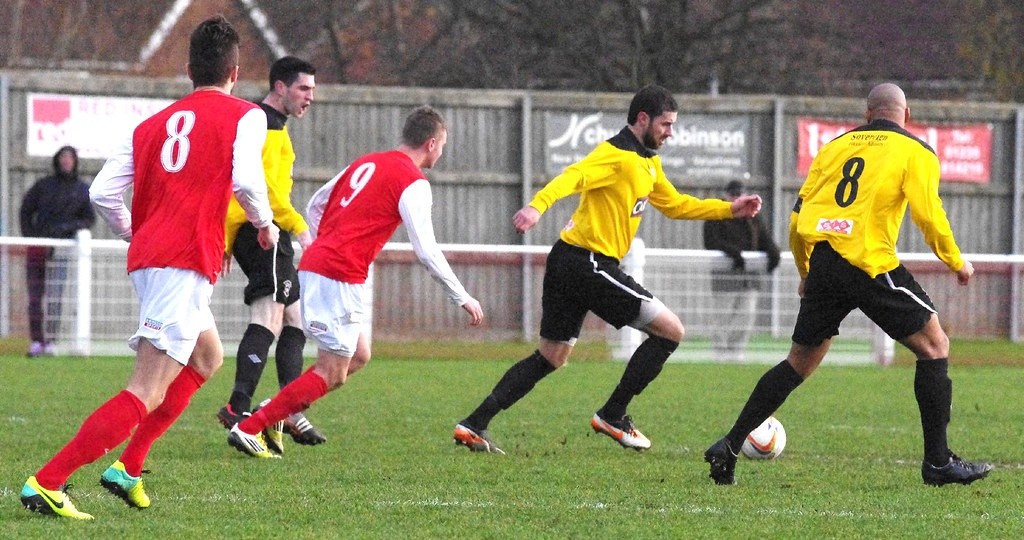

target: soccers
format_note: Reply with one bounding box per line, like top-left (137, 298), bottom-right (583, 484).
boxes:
top-left (740, 416), bottom-right (786, 460)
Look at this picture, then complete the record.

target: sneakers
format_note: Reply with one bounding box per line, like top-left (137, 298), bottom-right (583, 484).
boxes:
top-left (227, 423), bottom-right (272, 459)
top-left (252, 398), bottom-right (284, 453)
top-left (283, 411), bottom-right (327, 446)
top-left (591, 411), bottom-right (652, 451)
top-left (453, 421), bottom-right (500, 453)
top-left (20, 476), bottom-right (96, 522)
top-left (100, 459), bottom-right (151, 510)
top-left (704, 438), bottom-right (739, 487)
top-left (217, 404), bottom-right (253, 431)
top-left (921, 449), bottom-right (992, 488)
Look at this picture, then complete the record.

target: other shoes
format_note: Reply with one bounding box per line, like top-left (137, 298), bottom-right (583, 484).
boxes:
top-left (44, 341), bottom-right (58, 358)
top-left (26, 340), bottom-right (45, 356)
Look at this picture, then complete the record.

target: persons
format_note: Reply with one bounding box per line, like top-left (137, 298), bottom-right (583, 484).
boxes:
top-left (454, 84), bottom-right (762, 454)
top-left (20, 16), bottom-right (280, 519)
top-left (19, 146), bottom-right (97, 356)
top-left (228, 105), bottom-right (483, 458)
top-left (704, 179), bottom-right (781, 353)
top-left (705, 83), bottom-right (991, 487)
top-left (224, 56), bottom-right (327, 447)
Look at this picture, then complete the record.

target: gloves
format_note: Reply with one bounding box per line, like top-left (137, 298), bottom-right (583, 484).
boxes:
top-left (766, 247), bottom-right (779, 272)
top-left (731, 251), bottom-right (746, 271)
